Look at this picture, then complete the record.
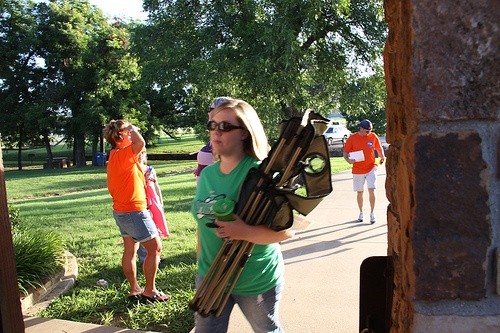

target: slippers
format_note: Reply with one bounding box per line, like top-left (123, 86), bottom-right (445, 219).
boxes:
top-left (141, 290), bottom-right (169, 301)
top-left (129, 290), bottom-right (144, 300)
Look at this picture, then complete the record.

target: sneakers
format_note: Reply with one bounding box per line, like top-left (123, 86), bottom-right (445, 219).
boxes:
top-left (358, 213), bottom-right (363, 221)
top-left (370, 213), bottom-right (376, 224)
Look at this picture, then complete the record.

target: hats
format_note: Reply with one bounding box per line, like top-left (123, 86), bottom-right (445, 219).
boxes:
top-left (356, 119), bottom-right (373, 129)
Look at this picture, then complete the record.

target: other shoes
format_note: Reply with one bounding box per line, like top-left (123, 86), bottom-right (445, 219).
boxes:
top-left (157, 269), bottom-right (161, 275)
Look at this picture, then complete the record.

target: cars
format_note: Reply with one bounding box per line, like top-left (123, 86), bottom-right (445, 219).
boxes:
top-left (323, 124), bottom-right (352, 147)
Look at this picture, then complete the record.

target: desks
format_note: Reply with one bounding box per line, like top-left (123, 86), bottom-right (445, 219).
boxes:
top-left (44, 157), bottom-right (68, 169)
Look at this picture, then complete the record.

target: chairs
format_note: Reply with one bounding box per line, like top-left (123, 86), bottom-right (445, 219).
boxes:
top-left (189, 110), bottom-right (332, 319)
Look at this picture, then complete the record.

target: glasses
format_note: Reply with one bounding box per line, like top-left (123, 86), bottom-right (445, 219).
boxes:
top-left (207, 121), bottom-right (244, 132)
top-left (207, 106), bottom-right (215, 111)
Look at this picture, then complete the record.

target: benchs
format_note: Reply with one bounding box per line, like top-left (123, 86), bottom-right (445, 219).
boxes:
top-left (44, 161), bottom-right (70, 168)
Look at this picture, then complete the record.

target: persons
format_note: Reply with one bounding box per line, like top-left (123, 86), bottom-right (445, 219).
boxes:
top-left (344, 120), bottom-right (385, 222)
top-left (102, 96), bottom-right (233, 302)
top-left (190, 98), bottom-right (296, 333)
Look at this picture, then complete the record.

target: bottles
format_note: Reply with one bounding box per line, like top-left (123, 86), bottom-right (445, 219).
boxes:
top-left (212, 198), bottom-right (234, 222)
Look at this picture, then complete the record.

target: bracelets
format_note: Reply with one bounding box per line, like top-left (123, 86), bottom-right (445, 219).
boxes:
top-left (381, 159), bottom-right (384, 161)
